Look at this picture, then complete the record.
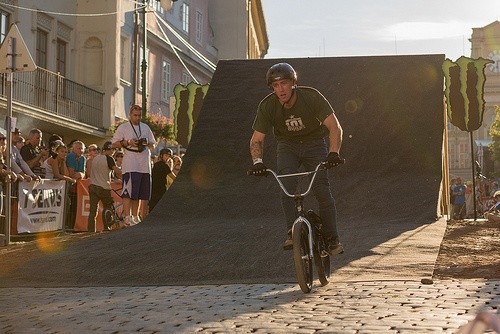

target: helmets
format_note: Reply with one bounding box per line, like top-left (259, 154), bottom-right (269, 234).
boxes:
top-left (160, 148), bottom-right (173, 156)
top-left (267, 63), bottom-right (297, 87)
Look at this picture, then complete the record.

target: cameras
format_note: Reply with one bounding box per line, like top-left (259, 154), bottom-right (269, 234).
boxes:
top-left (135, 137), bottom-right (149, 151)
top-left (48, 149), bottom-right (58, 159)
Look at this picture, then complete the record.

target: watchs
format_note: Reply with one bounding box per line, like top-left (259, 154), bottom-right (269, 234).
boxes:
top-left (253, 158), bottom-right (263, 165)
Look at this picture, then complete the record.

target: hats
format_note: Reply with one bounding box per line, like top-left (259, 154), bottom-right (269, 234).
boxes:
top-left (12, 128), bottom-right (21, 133)
top-left (0, 132), bottom-right (6, 139)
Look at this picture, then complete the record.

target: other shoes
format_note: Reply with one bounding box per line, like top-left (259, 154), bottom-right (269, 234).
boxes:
top-left (104, 225), bottom-right (111, 231)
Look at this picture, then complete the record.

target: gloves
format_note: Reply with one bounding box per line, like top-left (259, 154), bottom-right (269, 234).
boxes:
top-left (328, 152), bottom-right (340, 165)
top-left (252, 163), bottom-right (266, 176)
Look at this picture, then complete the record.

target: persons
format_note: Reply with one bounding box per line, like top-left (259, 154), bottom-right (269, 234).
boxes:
top-left (112, 105), bottom-right (156, 228)
top-left (0, 128), bottom-right (182, 234)
top-left (88, 140), bottom-right (122, 233)
top-left (250, 63), bottom-right (344, 255)
top-left (449, 177), bottom-right (500, 223)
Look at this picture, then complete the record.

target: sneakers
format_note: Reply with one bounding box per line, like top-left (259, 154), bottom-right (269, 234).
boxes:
top-left (283, 235), bottom-right (293, 249)
top-left (329, 240), bottom-right (344, 255)
top-left (125, 216), bottom-right (137, 226)
top-left (134, 216), bottom-right (141, 223)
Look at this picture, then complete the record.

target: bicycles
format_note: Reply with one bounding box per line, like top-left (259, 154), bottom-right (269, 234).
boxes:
top-left (254, 159), bottom-right (335, 292)
top-left (102, 182), bottom-right (127, 231)
